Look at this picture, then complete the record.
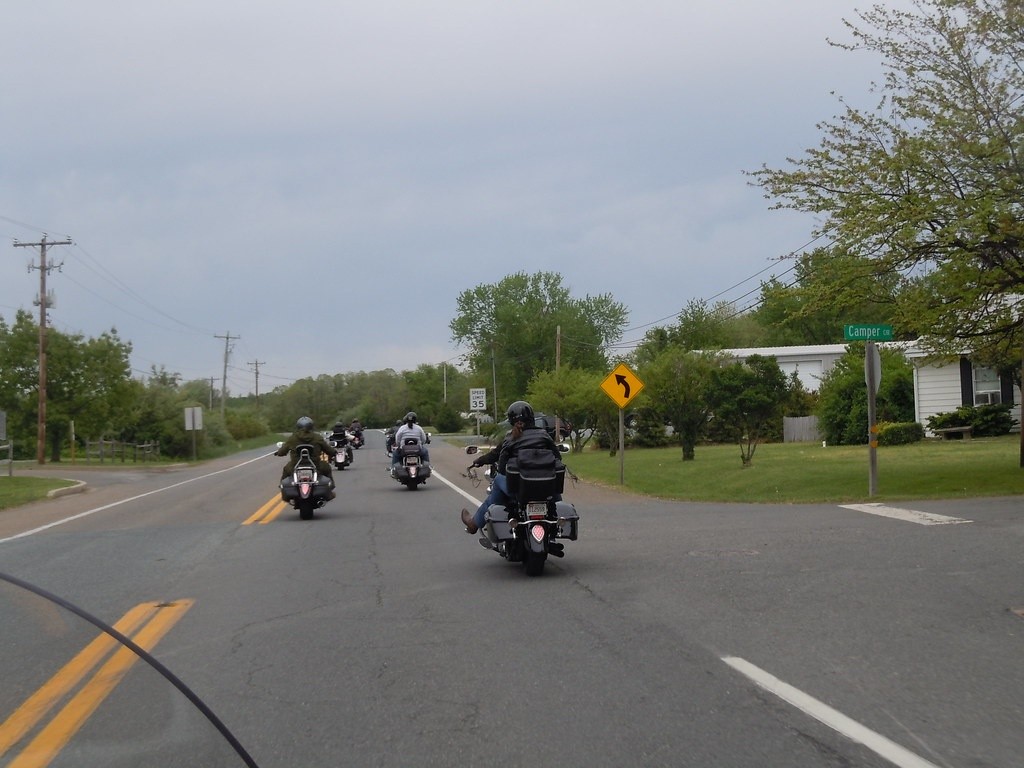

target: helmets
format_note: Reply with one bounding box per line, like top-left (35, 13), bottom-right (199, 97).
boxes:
top-left (507, 401), bottom-right (535, 427)
top-left (297, 416), bottom-right (314, 430)
top-left (334, 422), bottom-right (343, 427)
top-left (351, 417), bottom-right (359, 423)
top-left (402, 412), bottom-right (417, 423)
top-left (396, 420), bottom-right (403, 426)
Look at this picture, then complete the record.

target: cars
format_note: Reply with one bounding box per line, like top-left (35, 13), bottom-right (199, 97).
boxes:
top-left (499, 414), bottom-right (572, 443)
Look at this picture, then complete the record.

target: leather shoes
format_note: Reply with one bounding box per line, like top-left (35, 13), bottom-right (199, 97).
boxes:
top-left (461, 508), bottom-right (478, 535)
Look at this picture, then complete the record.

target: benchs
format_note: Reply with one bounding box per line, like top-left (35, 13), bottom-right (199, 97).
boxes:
top-left (932, 426), bottom-right (976, 441)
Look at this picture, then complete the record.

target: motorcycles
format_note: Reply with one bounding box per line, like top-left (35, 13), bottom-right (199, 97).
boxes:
top-left (346, 427), bottom-right (367, 449)
top-left (391, 432), bottom-right (433, 491)
top-left (383, 429), bottom-right (398, 457)
top-left (465, 442), bottom-right (579, 577)
top-left (325, 431), bottom-right (355, 469)
top-left (275, 441), bottom-right (338, 520)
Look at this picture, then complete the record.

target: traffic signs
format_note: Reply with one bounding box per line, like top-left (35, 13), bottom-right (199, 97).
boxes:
top-left (469, 388), bottom-right (486, 410)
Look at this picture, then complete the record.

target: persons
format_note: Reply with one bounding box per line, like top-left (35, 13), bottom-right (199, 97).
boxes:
top-left (385, 411), bottom-right (429, 468)
top-left (349, 418), bottom-right (364, 443)
top-left (277, 416), bottom-right (336, 483)
top-left (328, 421), bottom-right (355, 463)
top-left (460, 401), bottom-right (564, 536)
